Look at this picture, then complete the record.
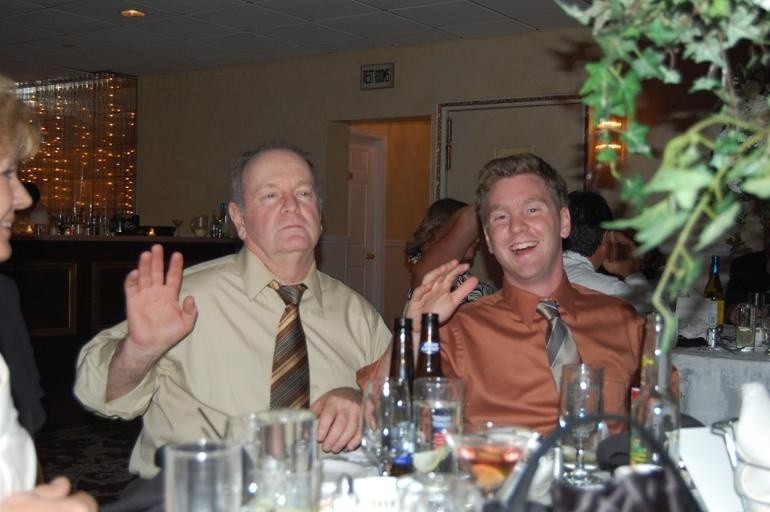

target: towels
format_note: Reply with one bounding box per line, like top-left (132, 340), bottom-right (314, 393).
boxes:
top-left (735, 382), bottom-right (770, 512)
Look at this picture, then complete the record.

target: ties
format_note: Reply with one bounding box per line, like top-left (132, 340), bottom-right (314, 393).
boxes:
top-left (269, 282), bottom-right (310, 410)
top-left (537, 299), bottom-right (584, 392)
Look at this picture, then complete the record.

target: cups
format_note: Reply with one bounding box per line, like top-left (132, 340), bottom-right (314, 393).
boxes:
top-left (732, 288), bottom-right (770, 356)
top-left (160, 438), bottom-right (246, 512)
top-left (190, 214), bottom-right (208, 240)
top-left (246, 410), bottom-right (322, 511)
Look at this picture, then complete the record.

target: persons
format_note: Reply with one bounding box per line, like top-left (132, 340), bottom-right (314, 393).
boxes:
top-left (15, 183), bottom-right (49, 224)
top-left (70, 137), bottom-right (393, 512)
top-left (558, 189), bottom-right (656, 316)
top-left (0, 85), bottom-right (101, 511)
top-left (403, 197), bottom-right (499, 319)
top-left (402, 151), bottom-right (681, 452)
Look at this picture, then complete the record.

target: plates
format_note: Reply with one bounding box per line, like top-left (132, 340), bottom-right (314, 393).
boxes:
top-left (734, 462), bottom-right (770, 506)
top-left (466, 423), bottom-right (538, 449)
top-left (336, 475), bottom-right (420, 512)
top-left (299, 455), bottom-right (363, 502)
top-left (732, 437), bottom-right (770, 470)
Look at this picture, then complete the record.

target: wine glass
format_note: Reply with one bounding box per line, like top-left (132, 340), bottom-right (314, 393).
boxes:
top-left (362, 377), bottom-right (470, 512)
top-left (557, 360), bottom-right (606, 486)
top-left (172, 213), bottom-right (185, 238)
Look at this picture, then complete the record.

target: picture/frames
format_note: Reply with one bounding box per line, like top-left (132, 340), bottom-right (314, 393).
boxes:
top-left (0, 261), bottom-right (77, 337)
top-left (91, 261), bottom-right (139, 334)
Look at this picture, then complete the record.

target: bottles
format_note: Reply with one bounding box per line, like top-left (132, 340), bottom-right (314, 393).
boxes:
top-left (378, 315), bottom-right (415, 479)
top-left (410, 312), bottom-right (453, 476)
top-left (630, 314), bottom-right (681, 469)
top-left (702, 255), bottom-right (726, 334)
top-left (32, 201), bottom-right (123, 237)
top-left (705, 326), bottom-right (718, 350)
top-left (211, 202), bottom-right (232, 239)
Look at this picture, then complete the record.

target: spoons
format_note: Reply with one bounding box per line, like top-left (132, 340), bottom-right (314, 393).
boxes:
top-left (708, 418), bottom-right (751, 511)
